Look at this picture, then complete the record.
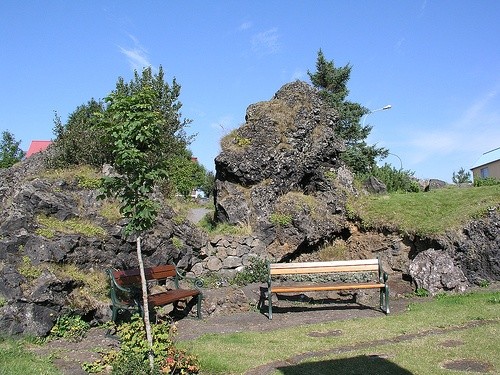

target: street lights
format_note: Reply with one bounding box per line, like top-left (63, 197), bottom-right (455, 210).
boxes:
top-left (361, 105), bottom-right (392, 130)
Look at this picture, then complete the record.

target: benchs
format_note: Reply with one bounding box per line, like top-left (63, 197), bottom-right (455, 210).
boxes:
top-left (105, 262), bottom-right (203, 337)
top-left (267, 256), bottom-right (391, 319)
